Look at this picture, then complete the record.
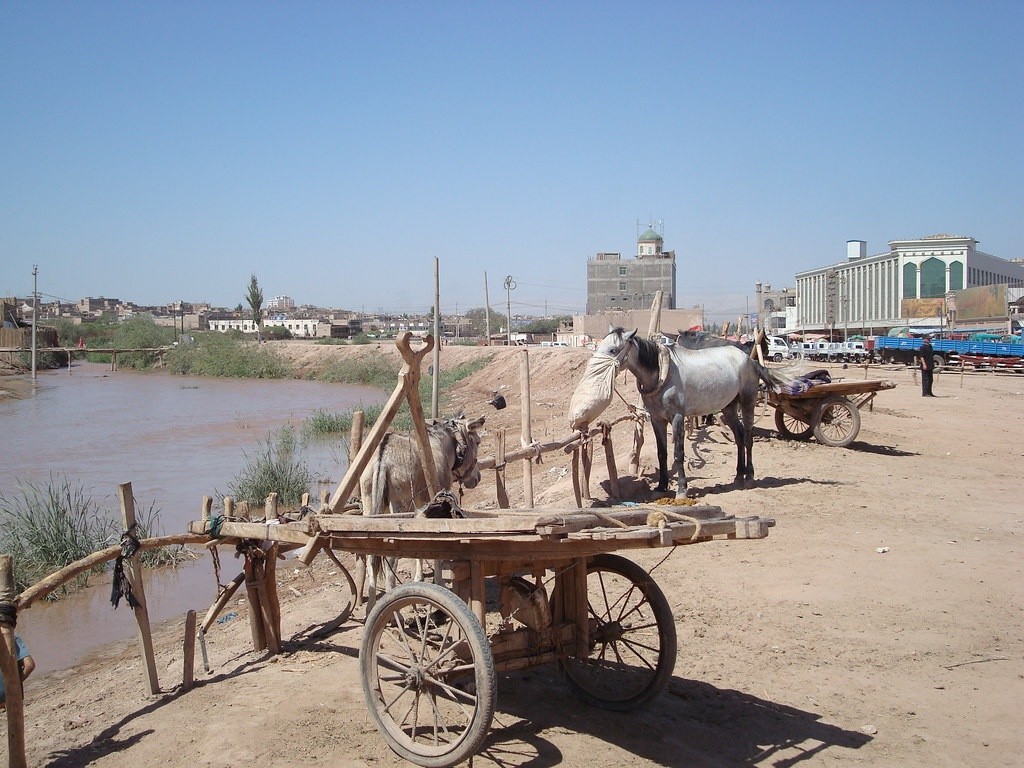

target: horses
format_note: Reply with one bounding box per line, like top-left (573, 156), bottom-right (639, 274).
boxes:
top-left (588, 325), bottom-right (770, 501)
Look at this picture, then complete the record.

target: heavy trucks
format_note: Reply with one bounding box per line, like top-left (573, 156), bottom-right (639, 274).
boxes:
top-left (874, 328), bottom-right (1024, 373)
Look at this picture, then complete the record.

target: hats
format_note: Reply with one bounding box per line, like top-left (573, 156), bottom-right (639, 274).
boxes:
top-left (922, 333), bottom-right (932, 339)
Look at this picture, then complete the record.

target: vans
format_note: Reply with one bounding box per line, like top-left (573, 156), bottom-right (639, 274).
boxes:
top-left (541, 342), bottom-right (568, 348)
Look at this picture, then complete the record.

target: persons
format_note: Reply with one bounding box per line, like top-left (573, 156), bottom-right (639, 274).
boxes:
top-left (919, 335), bottom-right (937, 397)
top-left (487, 390), bottom-right (506, 410)
top-left (78, 336), bottom-right (85, 348)
top-left (0, 637), bottom-right (36, 709)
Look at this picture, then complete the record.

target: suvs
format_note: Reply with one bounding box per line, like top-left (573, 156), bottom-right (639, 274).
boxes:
top-left (765, 337), bottom-right (789, 363)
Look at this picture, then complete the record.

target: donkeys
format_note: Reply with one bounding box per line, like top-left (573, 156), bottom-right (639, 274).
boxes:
top-left (360, 415), bottom-right (487, 620)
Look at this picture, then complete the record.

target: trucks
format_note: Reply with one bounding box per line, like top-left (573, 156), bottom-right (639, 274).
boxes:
top-left (790, 342), bottom-right (868, 363)
